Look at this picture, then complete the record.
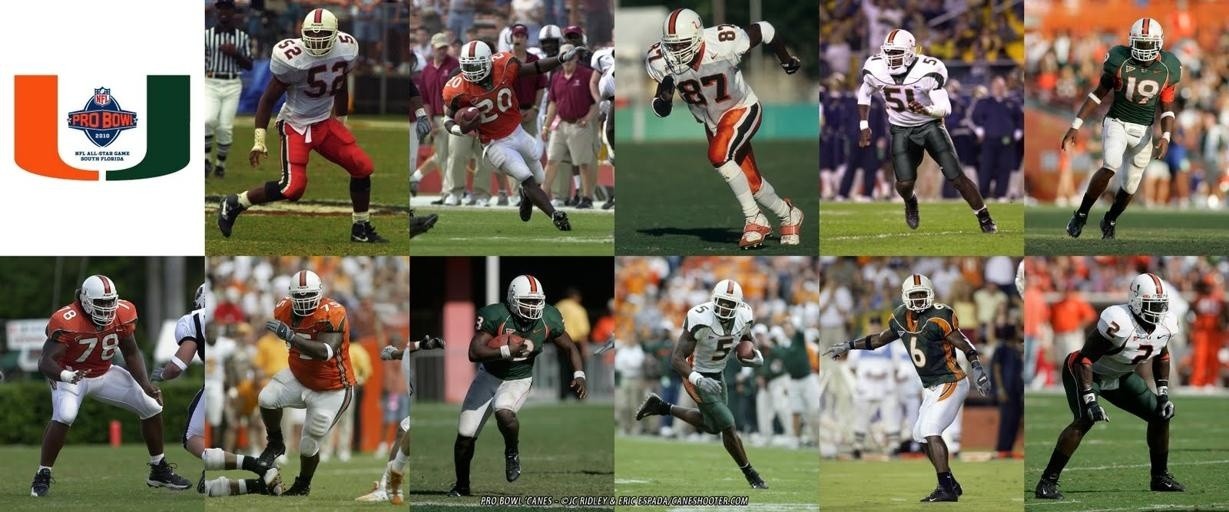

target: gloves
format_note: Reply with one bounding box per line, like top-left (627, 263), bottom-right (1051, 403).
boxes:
top-left (593, 336), bottom-right (615, 356)
top-left (381, 335), bottom-right (445, 361)
top-left (264, 320), bottom-right (295, 341)
top-left (780, 56), bottom-right (801, 75)
top-left (658, 76), bottom-right (675, 105)
top-left (416, 116), bottom-right (431, 137)
top-left (150, 368), bottom-right (164, 383)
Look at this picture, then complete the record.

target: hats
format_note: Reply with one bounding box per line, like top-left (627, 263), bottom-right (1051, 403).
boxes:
top-left (430, 24), bottom-right (582, 63)
top-left (214, 1), bottom-right (234, 7)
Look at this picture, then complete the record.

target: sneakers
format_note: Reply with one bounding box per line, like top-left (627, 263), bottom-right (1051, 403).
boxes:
top-left (920, 478), bottom-right (1184, 502)
top-left (745, 470), bottom-right (768, 490)
top-left (30, 468), bottom-right (51, 497)
top-left (635, 393), bottom-right (662, 421)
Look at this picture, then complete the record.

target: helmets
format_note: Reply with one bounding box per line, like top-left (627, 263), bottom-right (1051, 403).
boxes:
top-left (79, 270), bottom-right (322, 327)
top-left (879, 29), bottom-right (916, 75)
top-left (301, 8), bottom-right (338, 58)
top-left (507, 275), bottom-right (546, 321)
top-left (659, 8), bottom-right (703, 68)
top-left (1128, 273), bottom-right (1170, 325)
top-left (711, 280), bottom-right (744, 320)
top-left (901, 274), bottom-right (935, 313)
top-left (458, 40), bottom-right (493, 82)
top-left (1128, 18), bottom-right (1163, 61)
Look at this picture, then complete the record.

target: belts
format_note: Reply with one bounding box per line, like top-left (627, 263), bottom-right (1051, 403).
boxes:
top-left (208, 74), bottom-right (237, 79)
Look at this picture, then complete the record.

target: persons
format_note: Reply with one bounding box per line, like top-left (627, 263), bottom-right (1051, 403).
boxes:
top-left (410, 0), bottom-right (617, 240)
top-left (820, 1), bottom-right (1024, 234)
top-left (1025, 254), bottom-right (1228, 499)
top-left (204, 255), bottom-right (411, 510)
top-left (206, 2), bottom-right (409, 245)
top-left (1025, 0), bottom-right (1228, 237)
top-left (152, 282), bottom-right (204, 492)
top-left (32, 273), bottom-right (192, 498)
top-left (642, 8), bottom-right (805, 248)
top-left (410, 273), bottom-right (614, 497)
top-left (614, 257), bottom-right (818, 489)
top-left (819, 256), bottom-right (1025, 504)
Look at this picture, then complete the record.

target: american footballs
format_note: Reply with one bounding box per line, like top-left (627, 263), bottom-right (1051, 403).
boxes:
top-left (454, 107), bottom-right (480, 124)
top-left (488, 333), bottom-right (524, 348)
top-left (736, 332), bottom-right (774, 361)
